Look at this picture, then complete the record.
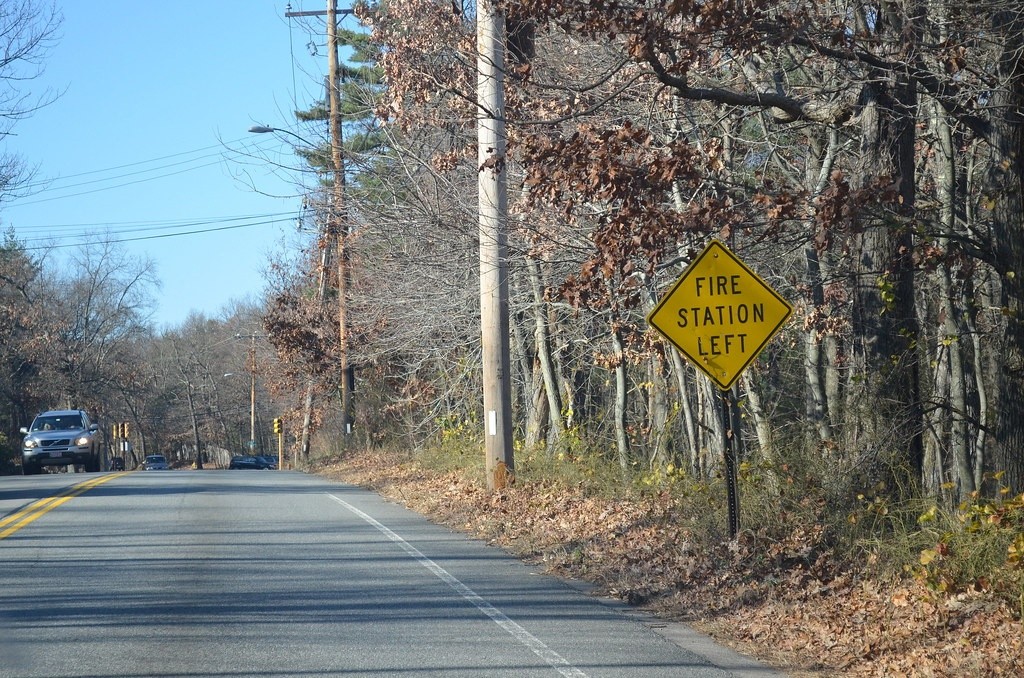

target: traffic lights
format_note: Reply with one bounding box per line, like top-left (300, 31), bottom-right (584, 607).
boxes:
top-left (273, 418), bottom-right (282, 434)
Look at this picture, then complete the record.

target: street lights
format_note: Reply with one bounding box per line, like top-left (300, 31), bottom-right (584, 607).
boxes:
top-left (247, 124), bottom-right (357, 440)
top-left (224, 373), bottom-right (256, 454)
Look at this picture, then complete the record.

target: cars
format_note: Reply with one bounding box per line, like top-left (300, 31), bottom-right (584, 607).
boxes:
top-left (110, 457), bottom-right (124, 471)
top-left (230, 454), bottom-right (278, 471)
top-left (20, 408), bottom-right (103, 476)
top-left (142, 455), bottom-right (168, 470)
